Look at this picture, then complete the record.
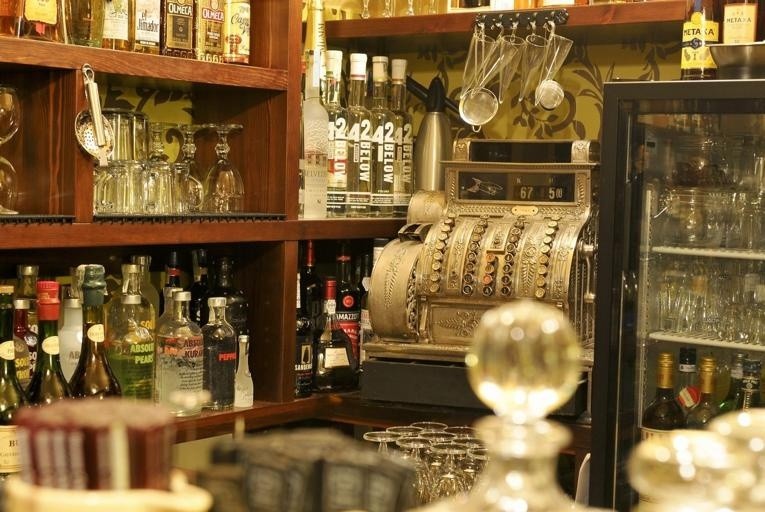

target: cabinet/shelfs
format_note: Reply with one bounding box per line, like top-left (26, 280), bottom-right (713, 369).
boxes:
top-left (592, 80), bottom-right (764, 510)
top-left (1, 0), bottom-right (765, 476)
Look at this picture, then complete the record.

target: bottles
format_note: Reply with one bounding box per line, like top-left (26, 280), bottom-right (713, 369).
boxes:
top-left (186, 248), bottom-right (209, 328)
top-left (301, 0), bottom-right (328, 100)
top-left (37, 282), bottom-right (59, 298)
top-left (316, 279), bottom-right (353, 393)
top-left (156, 291), bottom-right (204, 418)
top-left (0, 285), bottom-right (30, 390)
top-left (64, 0), bottom-right (105, 47)
top-left (337, 244), bottom-right (360, 368)
top-left (154, 287), bottom-right (184, 402)
top-left (68, 265), bottom-right (121, 399)
top-left (345, 53), bottom-right (372, 218)
top-left (735, 358), bottom-right (762, 408)
top-left (224, 0), bottom-right (251, 65)
top-left (195, 0), bottom-right (225, 63)
top-left (641, 352), bottom-right (686, 439)
top-left (57, 299), bottom-right (83, 382)
top-left (134, 0), bottom-right (161, 56)
top-left (201, 297), bottom-right (236, 411)
top-left (676, 363), bottom-right (730, 408)
top-left (234, 335), bottom-right (254, 408)
top-left (359, 255), bottom-right (375, 372)
top-left (325, 51), bottom-right (348, 219)
top-left (160, 0), bottom-right (196, 59)
top-left (104, 264), bottom-right (155, 400)
top-left (723, 352), bottom-right (748, 410)
top-left (681, 0), bottom-right (720, 79)
top-left (390, 59), bottom-right (413, 217)
top-left (132, 254), bottom-right (160, 316)
top-left (295, 272), bottom-right (314, 397)
top-left (304, 239), bottom-right (324, 329)
top-left (370, 56), bottom-right (396, 218)
top-left (14, 265), bottom-right (39, 335)
top-left (25, 298), bottom-right (74, 404)
top-left (159, 251), bottom-right (182, 317)
top-left (13, 299), bottom-right (38, 383)
top-left (102, 0), bottom-right (136, 51)
top-left (675, 347), bottom-right (698, 396)
top-left (25, 0), bottom-right (64, 42)
top-left (200, 256), bottom-right (249, 335)
top-left (1, 304), bottom-right (28, 482)
top-left (685, 354), bottom-right (720, 430)
top-left (302, 51), bottom-right (329, 219)
top-left (723, 0), bottom-right (758, 42)
top-left (0, 0), bottom-right (25, 38)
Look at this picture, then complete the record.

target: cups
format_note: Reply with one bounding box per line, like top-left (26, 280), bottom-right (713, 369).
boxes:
top-left (454, 439), bottom-right (483, 462)
top-left (386, 425), bottom-right (422, 456)
top-left (0, 84), bottom-right (20, 145)
top-left (171, 162), bottom-right (191, 213)
top-left (0, 157), bottom-right (20, 215)
top-left (470, 449), bottom-right (492, 475)
top-left (179, 124), bottom-right (214, 211)
top-left (411, 422), bottom-right (446, 430)
top-left (202, 123), bottom-right (243, 212)
top-left (396, 438), bottom-right (434, 506)
top-left (365, 432), bottom-right (400, 452)
top-left (445, 428), bottom-right (476, 438)
top-left (433, 445), bottom-right (467, 497)
top-left (95, 113), bottom-right (176, 213)
top-left (417, 431), bottom-right (456, 480)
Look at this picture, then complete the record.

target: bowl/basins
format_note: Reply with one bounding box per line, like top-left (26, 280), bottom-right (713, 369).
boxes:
top-left (707, 43), bottom-right (765, 78)
